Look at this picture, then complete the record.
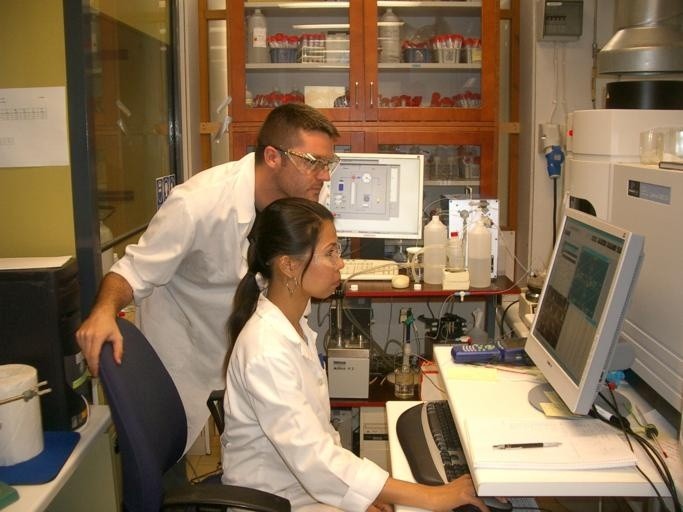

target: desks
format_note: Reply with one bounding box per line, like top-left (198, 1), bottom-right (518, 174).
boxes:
top-left (384, 343), bottom-right (682, 512)
top-left (0, 406), bottom-right (119, 512)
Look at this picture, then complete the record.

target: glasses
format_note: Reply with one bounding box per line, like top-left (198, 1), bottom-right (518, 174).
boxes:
top-left (276, 148), bottom-right (341, 174)
top-left (290, 243), bottom-right (342, 258)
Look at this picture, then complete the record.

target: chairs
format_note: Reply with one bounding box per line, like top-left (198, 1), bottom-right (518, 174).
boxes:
top-left (96, 318), bottom-right (291, 512)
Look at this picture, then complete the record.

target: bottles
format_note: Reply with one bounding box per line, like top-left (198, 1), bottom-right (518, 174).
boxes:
top-left (379, 8), bottom-right (403, 63)
top-left (448, 232), bottom-right (464, 268)
top-left (468, 221), bottom-right (491, 288)
top-left (247, 9), bottom-right (268, 64)
top-left (423, 215), bottom-right (447, 284)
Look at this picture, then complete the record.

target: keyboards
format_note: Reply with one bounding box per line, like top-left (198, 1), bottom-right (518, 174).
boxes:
top-left (338, 257), bottom-right (400, 281)
top-left (396, 400), bottom-right (470, 486)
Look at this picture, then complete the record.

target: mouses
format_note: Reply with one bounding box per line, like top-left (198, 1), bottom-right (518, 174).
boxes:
top-left (453, 496), bottom-right (513, 512)
top-left (390, 274), bottom-right (410, 289)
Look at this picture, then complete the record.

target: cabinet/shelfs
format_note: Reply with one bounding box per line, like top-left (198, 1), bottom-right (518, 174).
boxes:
top-left (309, 275), bottom-right (520, 408)
top-left (229, 123), bottom-right (501, 261)
top-left (226, 0), bottom-right (500, 121)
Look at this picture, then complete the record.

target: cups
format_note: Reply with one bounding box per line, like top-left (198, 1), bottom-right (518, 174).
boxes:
top-left (640, 131), bottom-right (665, 166)
top-left (393, 369), bottom-right (414, 399)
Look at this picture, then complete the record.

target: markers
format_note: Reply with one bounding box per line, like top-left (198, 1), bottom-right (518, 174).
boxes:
top-left (592, 405), bottom-right (624, 429)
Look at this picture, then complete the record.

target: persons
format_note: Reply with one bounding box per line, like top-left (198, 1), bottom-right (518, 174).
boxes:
top-left (219, 197), bottom-right (507, 512)
top-left (75, 105), bottom-right (342, 466)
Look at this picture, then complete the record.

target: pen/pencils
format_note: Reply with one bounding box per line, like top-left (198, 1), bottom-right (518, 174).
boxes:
top-left (493, 443), bottom-right (559, 449)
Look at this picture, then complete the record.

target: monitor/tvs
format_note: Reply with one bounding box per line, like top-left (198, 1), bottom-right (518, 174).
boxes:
top-left (523, 207), bottom-right (646, 420)
top-left (318, 153), bottom-right (425, 256)
